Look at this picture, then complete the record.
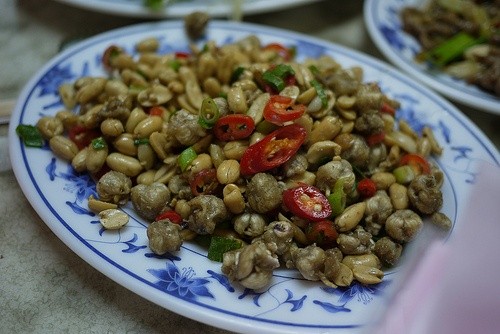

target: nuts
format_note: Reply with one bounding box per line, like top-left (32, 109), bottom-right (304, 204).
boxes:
top-left (39, 36), bottom-right (444, 288)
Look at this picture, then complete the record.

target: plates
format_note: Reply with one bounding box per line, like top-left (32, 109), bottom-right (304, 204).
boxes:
top-left (8, 19), bottom-right (500, 334)
top-left (56, 0), bottom-right (321, 19)
top-left (363, 0), bottom-right (500, 115)
